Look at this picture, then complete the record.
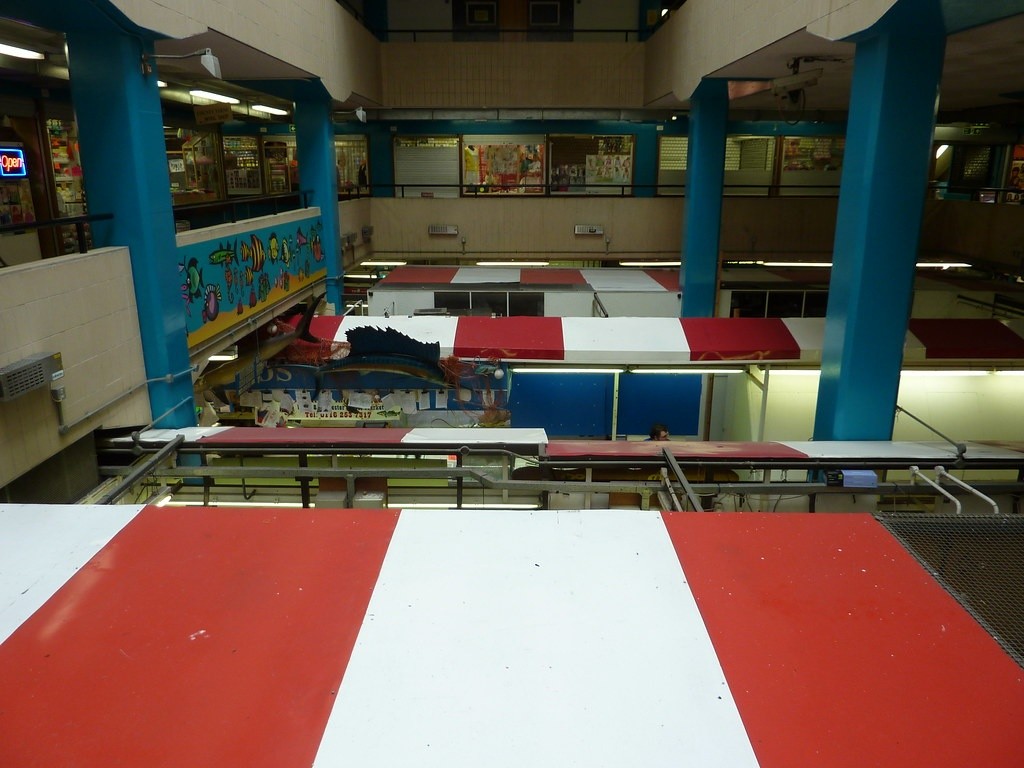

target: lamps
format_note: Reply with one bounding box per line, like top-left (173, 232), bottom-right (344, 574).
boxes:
top-left (251, 102), bottom-right (289, 116)
top-left (189, 87), bottom-right (240, 105)
top-left (143, 47), bottom-right (222, 80)
top-left (0, 38), bottom-right (46, 60)
top-left (327, 106), bottom-right (366, 124)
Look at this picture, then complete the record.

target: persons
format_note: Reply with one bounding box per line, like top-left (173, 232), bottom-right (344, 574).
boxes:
top-left (0, 210), bottom-right (10, 224)
top-left (12, 206), bottom-right (22, 224)
top-left (623, 158), bottom-right (630, 178)
top-left (643, 424), bottom-right (671, 441)
top-left (1007, 194), bottom-right (1013, 202)
top-left (550, 169), bottom-right (559, 185)
top-left (24, 207), bottom-right (33, 222)
top-left (484, 171), bottom-right (489, 182)
top-left (615, 156), bottom-right (621, 172)
top-left (589, 155), bottom-right (611, 175)
top-left (1009, 163), bottom-right (1024, 186)
top-left (528, 154), bottom-right (541, 170)
top-left (1020, 194), bottom-right (1024, 205)
top-left (1014, 195), bottom-right (1019, 202)
top-left (358, 160), bottom-right (367, 192)
top-left (0, 184), bottom-right (20, 205)
